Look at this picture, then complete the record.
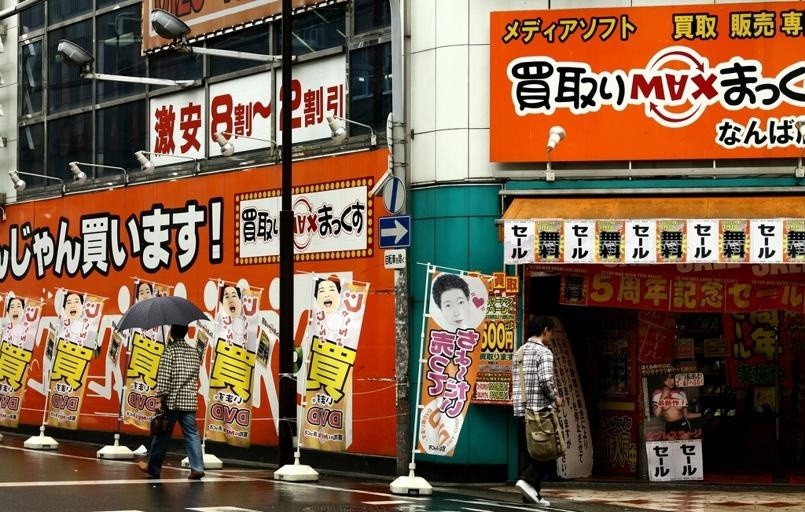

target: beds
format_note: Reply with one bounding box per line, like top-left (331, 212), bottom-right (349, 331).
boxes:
top-left (111, 295), bottom-right (212, 349)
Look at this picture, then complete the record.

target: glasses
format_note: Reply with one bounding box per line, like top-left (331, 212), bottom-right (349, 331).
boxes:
top-left (541, 125), bottom-right (567, 183)
top-left (324, 114), bottom-right (380, 149)
top-left (213, 130), bottom-right (280, 163)
top-left (791, 114), bottom-right (805, 180)
top-left (68, 160), bottom-right (130, 187)
top-left (54, 37), bottom-right (206, 92)
top-left (148, 7), bottom-right (296, 66)
top-left (8, 168), bottom-right (66, 194)
top-left (133, 148), bottom-right (198, 178)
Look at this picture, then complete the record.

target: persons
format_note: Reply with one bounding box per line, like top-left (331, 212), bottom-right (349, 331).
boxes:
top-left (62, 292), bottom-right (83, 320)
top-left (137, 325), bottom-right (207, 481)
top-left (296, 275), bottom-right (344, 404)
top-left (434, 273), bottom-right (472, 330)
top-left (5, 297), bottom-right (26, 324)
top-left (220, 284), bottom-right (245, 320)
top-left (509, 314), bottom-right (564, 508)
top-left (652, 375), bottom-right (694, 431)
top-left (135, 279), bottom-right (153, 304)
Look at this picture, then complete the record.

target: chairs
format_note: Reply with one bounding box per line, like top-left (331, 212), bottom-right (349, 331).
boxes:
top-left (515, 478), bottom-right (551, 507)
top-left (138, 459), bottom-right (163, 477)
top-left (187, 469), bottom-right (206, 478)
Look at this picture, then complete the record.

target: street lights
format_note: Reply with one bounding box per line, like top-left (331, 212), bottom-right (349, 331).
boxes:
top-left (524, 407), bottom-right (568, 461)
